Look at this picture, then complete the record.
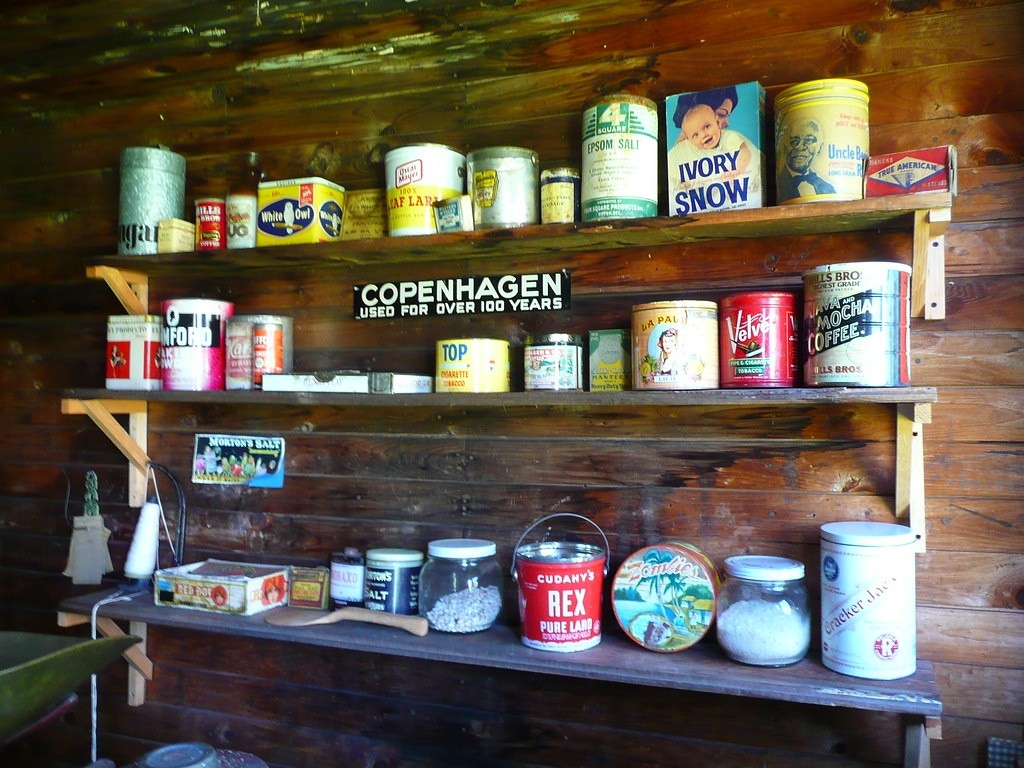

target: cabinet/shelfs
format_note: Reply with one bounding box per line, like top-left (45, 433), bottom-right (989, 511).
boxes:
top-left (62, 190), bottom-right (953, 768)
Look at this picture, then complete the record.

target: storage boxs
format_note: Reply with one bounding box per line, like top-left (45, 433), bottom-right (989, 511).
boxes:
top-left (262, 373), bottom-right (431, 394)
top-left (864, 145), bottom-right (958, 198)
top-left (288, 569), bottom-right (329, 608)
top-left (154, 560), bottom-right (291, 616)
top-left (589, 329), bottom-right (633, 391)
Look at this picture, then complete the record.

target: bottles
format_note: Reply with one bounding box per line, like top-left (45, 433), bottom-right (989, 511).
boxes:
top-left (538, 166), bottom-right (582, 226)
top-left (364, 547), bottom-right (426, 616)
top-left (105, 314), bottom-right (164, 392)
top-left (330, 552), bottom-right (366, 609)
top-left (418, 537), bottom-right (502, 635)
top-left (227, 150), bottom-right (267, 248)
top-left (715, 555), bottom-right (813, 669)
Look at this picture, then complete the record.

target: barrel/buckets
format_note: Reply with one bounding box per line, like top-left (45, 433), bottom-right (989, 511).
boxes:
top-left (509, 513), bottom-right (611, 653)
top-left (117, 139), bottom-right (188, 257)
top-left (818, 521), bottom-right (917, 681)
top-left (384, 142), bottom-right (471, 236)
top-left (630, 299), bottom-right (722, 392)
top-left (581, 93), bottom-right (658, 225)
top-left (466, 145), bottom-right (540, 231)
top-left (773, 77), bottom-right (878, 204)
top-left (800, 261), bottom-right (914, 390)
top-left (160, 297), bottom-right (236, 392)
top-left (433, 337), bottom-right (513, 393)
top-left (225, 314), bottom-right (295, 391)
top-left (718, 291), bottom-right (801, 389)
top-left (523, 332), bottom-right (585, 393)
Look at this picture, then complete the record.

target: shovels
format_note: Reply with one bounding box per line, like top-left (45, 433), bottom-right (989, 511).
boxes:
top-left (264, 606), bottom-right (429, 638)
